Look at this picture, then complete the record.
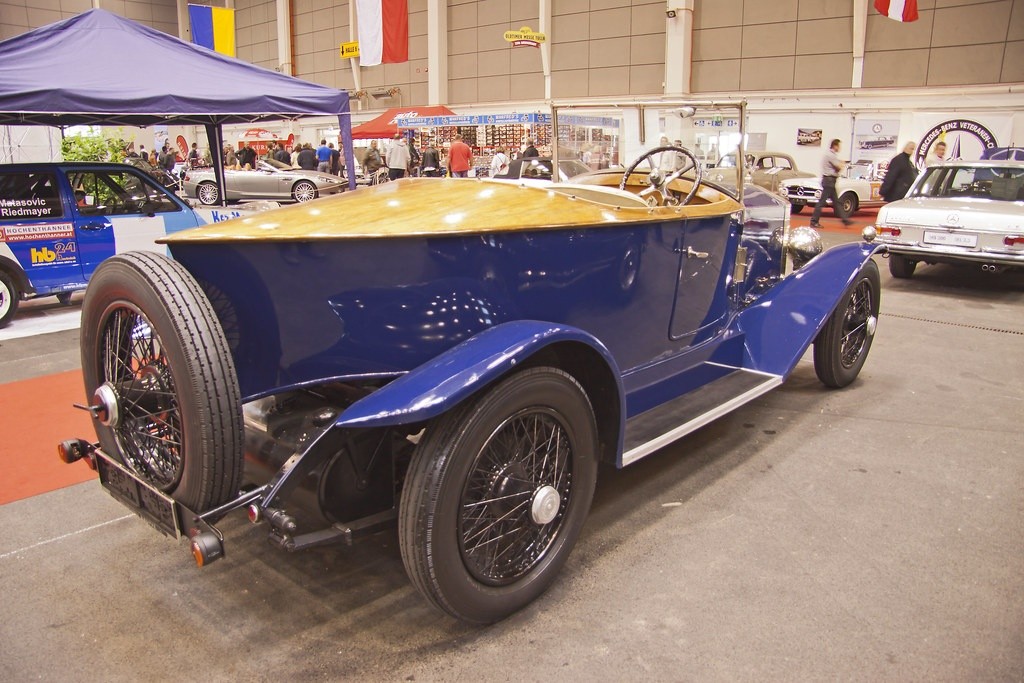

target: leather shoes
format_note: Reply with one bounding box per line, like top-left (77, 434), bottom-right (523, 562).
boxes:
top-left (810, 220), bottom-right (823, 228)
top-left (843, 220), bottom-right (855, 227)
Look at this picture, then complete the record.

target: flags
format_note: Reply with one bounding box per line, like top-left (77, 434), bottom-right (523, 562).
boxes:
top-left (873, 0), bottom-right (919, 23)
top-left (356, 0), bottom-right (408, 66)
top-left (188, 5), bottom-right (235, 58)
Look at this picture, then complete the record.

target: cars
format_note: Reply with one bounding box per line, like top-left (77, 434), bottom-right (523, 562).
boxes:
top-left (873, 159), bottom-right (1024, 279)
top-left (336, 150), bottom-right (387, 187)
top-left (493, 156), bottom-right (592, 183)
top-left (54, 137), bottom-right (883, 630)
top-left (703, 149), bottom-right (818, 215)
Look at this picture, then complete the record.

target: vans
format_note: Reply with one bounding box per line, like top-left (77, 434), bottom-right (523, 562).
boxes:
top-left (0, 162), bottom-right (212, 330)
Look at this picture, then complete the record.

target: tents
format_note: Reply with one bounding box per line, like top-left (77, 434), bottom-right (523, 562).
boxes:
top-left (0, 7), bottom-right (356, 208)
top-left (337, 105), bottom-right (459, 141)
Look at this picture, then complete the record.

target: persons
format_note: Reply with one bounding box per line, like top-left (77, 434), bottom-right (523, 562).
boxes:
top-left (523, 138), bottom-right (538, 157)
top-left (420, 142), bottom-right (439, 177)
top-left (880, 141), bottom-right (918, 201)
top-left (187, 143), bottom-right (199, 166)
top-left (925, 141), bottom-right (946, 192)
top-left (490, 146), bottom-right (510, 175)
top-left (447, 134), bottom-right (473, 177)
top-left (204, 139), bottom-right (256, 169)
top-left (810, 139), bottom-right (854, 229)
top-left (266, 139), bottom-right (346, 195)
top-left (121, 143), bottom-right (176, 174)
top-left (385, 134), bottom-right (411, 181)
top-left (657, 137), bottom-right (687, 172)
top-left (362, 139), bottom-right (384, 186)
top-left (407, 138), bottom-right (419, 177)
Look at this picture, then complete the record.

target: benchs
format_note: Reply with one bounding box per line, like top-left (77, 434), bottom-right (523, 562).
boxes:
top-left (486, 177), bottom-right (648, 208)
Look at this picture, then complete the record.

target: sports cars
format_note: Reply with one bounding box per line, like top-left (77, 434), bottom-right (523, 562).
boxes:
top-left (183, 157), bottom-right (349, 207)
top-left (777, 158), bottom-right (889, 220)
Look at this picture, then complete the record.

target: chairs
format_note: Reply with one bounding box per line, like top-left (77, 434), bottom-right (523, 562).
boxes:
top-left (231, 162), bottom-right (252, 171)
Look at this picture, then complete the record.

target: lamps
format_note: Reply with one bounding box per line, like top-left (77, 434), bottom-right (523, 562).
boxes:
top-left (348, 89), bottom-right (368, 111)
top-left (371, 87), bottom-right (402, 107)
